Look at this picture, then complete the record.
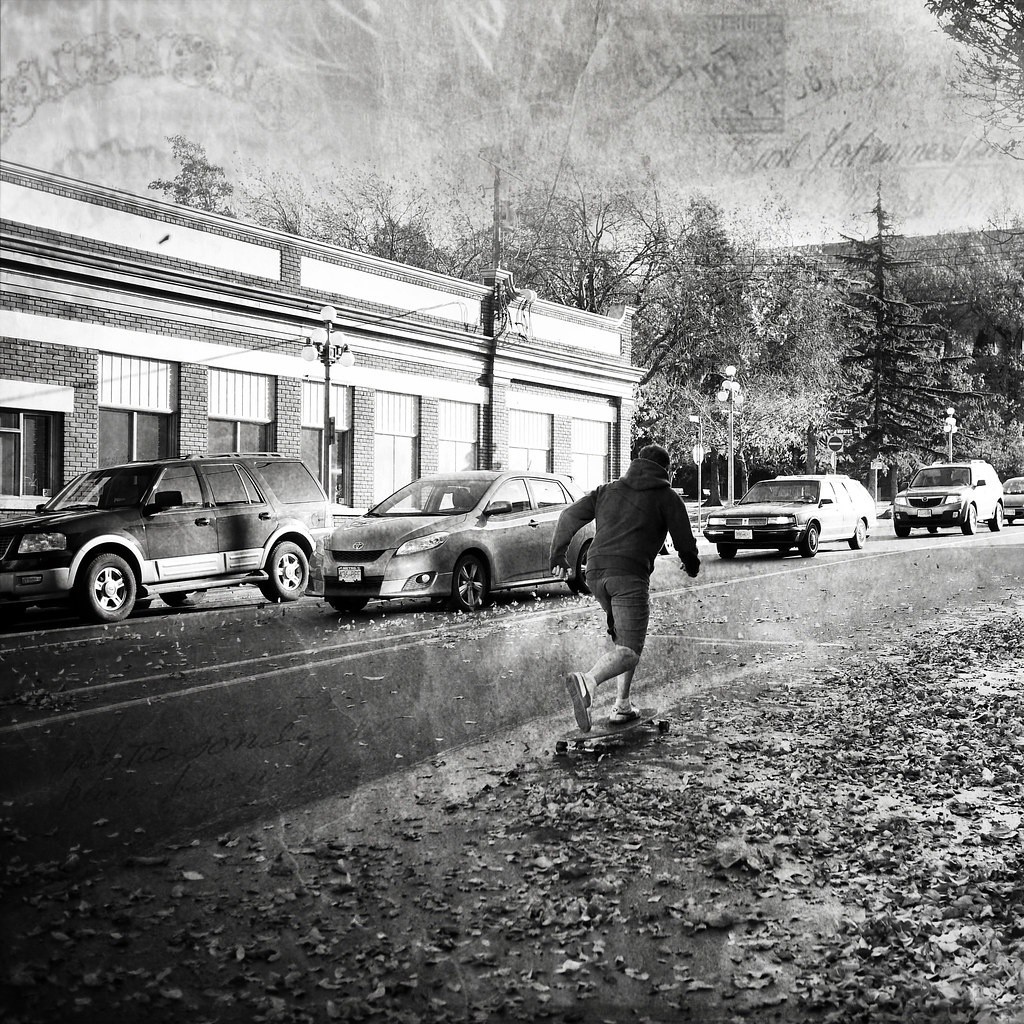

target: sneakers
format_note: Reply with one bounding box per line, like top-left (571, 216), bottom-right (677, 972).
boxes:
top-left (566, 670), bottom-right (592, 732)
top-left (609, 705), bottom-right (642, 723)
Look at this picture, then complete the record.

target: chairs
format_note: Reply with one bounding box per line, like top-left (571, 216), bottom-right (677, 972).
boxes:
top-left (453, 488), bottom-right (471, 511)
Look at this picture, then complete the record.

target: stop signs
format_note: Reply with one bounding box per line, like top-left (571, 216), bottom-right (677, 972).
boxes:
top-left (827, 436), bottom-right (844, 453)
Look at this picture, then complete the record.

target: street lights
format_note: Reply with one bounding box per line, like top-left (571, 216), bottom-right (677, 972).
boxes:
top-left (303, 307), bottom-right (356, 504)
top-left (943, 406), bottom-right (959, 464)
top-left (717, 365), bottom-right (744, 506)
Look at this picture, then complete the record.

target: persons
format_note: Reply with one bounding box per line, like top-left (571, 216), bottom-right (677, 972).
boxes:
top-left (551, 445), bottom-right (701, 733)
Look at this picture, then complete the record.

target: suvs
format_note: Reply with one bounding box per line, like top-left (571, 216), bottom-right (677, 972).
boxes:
top-left (703, 473), bottom-right (876, 559)
top-left (891, 459), bottom-right (1003, 538)
top-left (1, 451), bottom-right (336, 623)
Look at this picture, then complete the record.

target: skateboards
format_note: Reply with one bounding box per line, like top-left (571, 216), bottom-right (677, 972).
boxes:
top-left (556, 706), bottom-right (670, 757)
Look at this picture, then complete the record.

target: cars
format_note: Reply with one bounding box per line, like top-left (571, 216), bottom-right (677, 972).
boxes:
top-left (1001, 475), bottom-right (1024, 519)
top-left (304, 467), bottom-right (598, 617)
